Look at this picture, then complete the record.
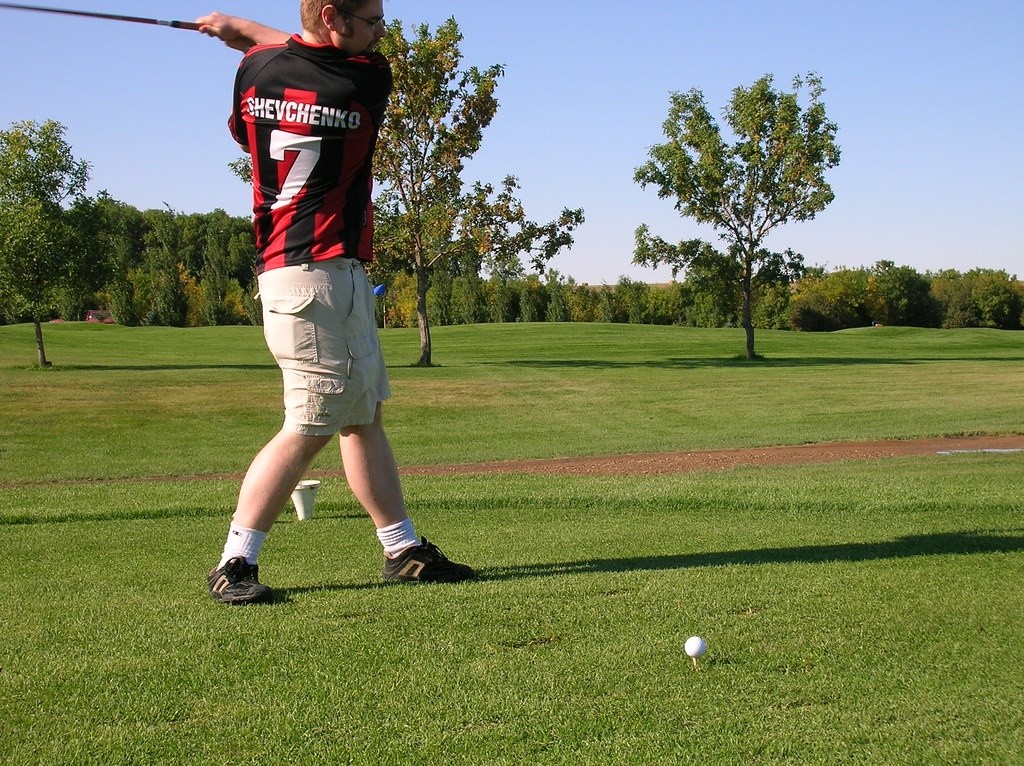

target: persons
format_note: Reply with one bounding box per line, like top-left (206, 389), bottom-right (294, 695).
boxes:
top-left (196, 0), bottom-right (474, 606)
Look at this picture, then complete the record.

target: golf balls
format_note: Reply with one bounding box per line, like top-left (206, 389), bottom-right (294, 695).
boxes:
top-left (684, 635), bottom-right (706, 658)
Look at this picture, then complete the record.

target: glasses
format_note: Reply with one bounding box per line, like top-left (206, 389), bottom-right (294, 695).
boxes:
top-left (338, 11), bottom-right (387, 26)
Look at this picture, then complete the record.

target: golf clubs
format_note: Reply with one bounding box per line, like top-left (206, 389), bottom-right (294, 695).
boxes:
top-left (1, 2), bottom-right (203, 31)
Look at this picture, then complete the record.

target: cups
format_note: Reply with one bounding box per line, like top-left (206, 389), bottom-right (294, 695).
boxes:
top-left (290, 480), bottom-right (321, 521)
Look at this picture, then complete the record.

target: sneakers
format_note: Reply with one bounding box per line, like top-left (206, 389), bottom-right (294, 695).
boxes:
top-left (208, 556), bottom-right (273, 605)
top-left (376, 534), bottom-right (475, 584)
